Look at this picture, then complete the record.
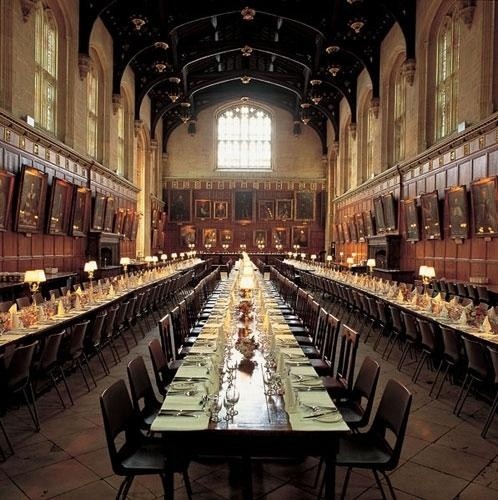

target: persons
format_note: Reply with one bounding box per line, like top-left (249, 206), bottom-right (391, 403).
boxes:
top-left (21, 182), bottom-right (38, 226)
top-left (273, 235), bottom-right (280, 245)
top-left (475, 188), bottom-right (496, 234)
top-left (172, 194), bottom-right (187, 221)
top-left (52, 192), bottom-right (63, 231)
top-left (449, 197), bottom-right (467, 235)
top-left (407, 206), bottom-right (418, 238)
top-left (297, 229), bottom-right (308, 247)
top-left (200, 203), bottom-right (209, 216)
top-left (236, 192), bottom-right (251, 220)
top-left (0, 179), bottom-right (6, 228)
top-left (255, 233), bottom-right (264, 243)
top-left (276, 231), bottom-right (284, 245)
top-left (215, 203), bottom-right (225, 216)
top-left (73, 198), bottom-right (83, 231)
top-left (423, 201), bottom-right (440, 237)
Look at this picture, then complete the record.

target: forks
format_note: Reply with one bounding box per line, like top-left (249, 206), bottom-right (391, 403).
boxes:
top-left (302, 403), bottom-right (337, 411)
top-left (165, 391), bottom-right (195, 396)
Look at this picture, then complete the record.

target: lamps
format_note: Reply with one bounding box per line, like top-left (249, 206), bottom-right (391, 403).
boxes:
top-left (293, 253), bottom-right (298, 260)
top-left (419, 265), bottom-right (436, 308)
top-left (120, 257), bottom-right (132, 288)
top-left (24, 268), bottom-right (47, 326)
top-left (287, 252), bottom-right (293, 260)
top-left (222, 244), bottom-right (230, 253)
top-left (188, 244), bottom-right (195, 251)
top-left (275, 244), bottom-right (282, 253)
top-left (293, 244), bottom-right (300, 257)
top-left (161, 254), bottom-right (168, 265)
top-left (241, 46), bottom-right (252, 56)
top-left (258, 245), bottom-right (265, 252)
top-left (366, 259), bottom-right (376, 285)
top-left (83, 260), bottom-right (100, 306)
top-left (130, 15), bottom-right (149, 31)
top-left (346, 256), bottom-right (353, 274)
top-left (309, 88), bottom-right (324, 106)
top-left (180, 252), bottom-right (185, 263)
top-left (168, 76), bottom-right (180, 85)
top-left (301, 253), bottom-right (306, 262)
top-left (193, 250), bottom-right (196, 259)
top-left (327, 256), bottom-right (332, 268)
top-left (204, 244), bottom-right (212, 253)
top-left (326, 46), bottom-right (340, 55)
top-left (186, 251), bottom-right (192, 261)
top-left (241, 7), bottom-right (255, 19)
top-left (241, 75), bottom-right (252, 85)
top-left (180, 102), bottom-right (190, 109)
top-left (348, 18), bottom-right (366, 33)
top-left (171, 253), bottom-right (177, 264)
top-left (310, 255), bottom-right (317, 268)
top-left (145, 256), bottom-right (153, 270)
top-left (310, 254), bottom-right (317, 266)
top-left (190, 250), bottom-right (193, 260)
top-left (155, 41), bottom-right (169, 50)
top-left (327, 65), bottom-right (341, 75)
top-left (152, 255), bottom-right (158, 269)
top-left (153, 61), bottom-right (169, 72)
top-left (240, 244), bottom-right (247, 252)
top-left (167, 91), bottom-right (180, 102)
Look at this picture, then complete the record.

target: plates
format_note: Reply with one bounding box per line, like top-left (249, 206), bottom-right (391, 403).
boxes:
top-left (312, 411), bottom-right (342, 422)
top-left (170, 382), bottom-right (196, 390)
top-left (184, 356), bottom-right (206, 362)
top-left (297, 374), bottom-right (323, 386)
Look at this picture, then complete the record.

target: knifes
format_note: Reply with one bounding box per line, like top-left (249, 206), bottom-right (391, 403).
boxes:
top-left (175, 377), bottom-right (208, 379)
top-left (294, 389), bottom-right (326, 391)
top-left (158, 413), bottom-right (195, 417)
top-left (161, 410), bottom-right (204, 413)
top-left (293, 386), bottom-right (325, 388)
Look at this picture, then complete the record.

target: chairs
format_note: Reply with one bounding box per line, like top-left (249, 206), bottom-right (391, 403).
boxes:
top-left (320, 379), bottom-right (413, 499)
top-left (148, 339), bottom-right (178, 398)
top-left (311, 313), bottom-right (341, 378)
top-left (66, 320), bottom-right (97, 393)
top-left (299, 114), bottom-right (311, 123)
top-left (137, 292), bottom-right (147, 340)
top-left (327, 281), bottom-right (342, 311)
top-left (185, 295), bottom-right (203, 336)
top-left (411, 318), bottom-right (439, 384)
top-left (100, 379), bottom-right (193, 500)
top-left (396, 311), bottom-right (421, 372)
top-left (333, 282), bottom-right (348, 316)
top-left (275, 257), bottom-right (313, 344)
top-left (457, 282), bottom-right (466, 298)
top-left (181, 257), bottom-right (229, 308)
top-left (446, 281), bottom-right (455, 298)
top-left (300, 102), bottom-right (311, 111)
top-left (365, 295), bottom-right (393, 344)
top-left (281, 283), bottom-right (298, 314)
top-left (175, 277), bottom-right (181, 303)
top-left (73, 283), bottom-right (82, 291)
top-left (431, 278), bottom-right (438, 290)
top-left (466, 284), bottom-right (478, 302)
top-left (188, 290), bottom-right (207, 327)
top-left (381, 305), bottom-right (406, 362)
top-left (193, 285), bottom-right (210, 320)
top-left (150, 283), bottom-right (161, 325)
top-left (312, 356), bottom-right (380, 489)
top-left (18, 297), bottom-right (30, 306)
top-left (475, 285), bottom-right (490, 305)
top-left (83, 282), bottom-right (89, 288)
top-left (357, 292), bottom-right (379, 335)
top-left (452, 335), bottom-right (493, 415)
top-left (62, 286), bottom-right (70, 296)
top-left (168, 306), bottom-right (191, 360)
top-left (290, 295), bottom-right (314, 336)
top-left (169, 278), bottom-right (176, 309)
top-left (269, 253), bottom-right (326, 299)
top-left (50, 288), bottom-right (60, 297)
top-left (178, 300), bottom-right (197, 346)
top-left (301, 308), bottom-right (329, 359)
top-left (143, 288), bottom-right (152, 332)
top-left (161, 281), bottom-right (170, 314)
top-left (285, 287), bottom-right (308, 326)
top-left (339, 284), bottom-right (358, 319)
top-left (32, 329), bottom-right (76, 410)
top-left (344, 286), bottom-right (362, 325)
top-left (429, 325), bottom-right (464, 400)
top-left (374, 299), bottom-right (394, 352)
top-left (351, 289), bottom-right (370, 329)
top-left (308, 78), bottom-right (321, 87)
top-left (439, 280), bottom-right (446, 292)
top-left (86, 311), bottom-right (109, 376)
top-left (148, 339), bottom-right (175, 395)
top-left (33, 291), bottom-right (43, 302)
top-left (3, 340), bottom-right (42, 455)
top-left (107, 306), bottom-right (122, 367)
top-left (127, 356), bottom-right (162, 437)
top-left (196, 256), bottom-right (296, 287)
top-left (116, 299), bottom-right (138, 353)
top-left (320, 325), bottom-right (360, 400)
top-left (127, 296), bottom-right (141, 346)
top-left (294, 301), bottom-right (320, 344)
top-left (0, 302), bottom-right (15, 311)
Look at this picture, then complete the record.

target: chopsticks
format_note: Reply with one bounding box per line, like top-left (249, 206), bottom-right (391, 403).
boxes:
top-left (303, 411), bottom-right (339, 419)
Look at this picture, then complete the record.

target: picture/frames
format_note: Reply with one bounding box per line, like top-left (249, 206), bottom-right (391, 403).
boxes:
top-left (469, 175), bottom-right (498, 241)
top-left (214, 201), bottom-right (228, 220)
top-left (168, 189), bottom-right (192, 224)
top-left (195, 200), bottom-right (211, 220)
top-left (202, 227), bottom-right (217, 247)
top-left (104, 197), bottom-right (115, 233)
top-left (253, 229), bottom-right (267, 248)
top-left (113, 209), bottom-right (124, 235)
top-left (349, 217), bottom-right (358, 243)
top-left (91, 194), bottom-right (106, 230)
top-left (131, 214), bottom-right (137, 240)
top-left (259, 199), bottom-right (274, 221)
top-left (0, 171), bottom-right (15, 234)
top-left (362, 209), bottom-right (375, 235)
top-left (291, 225), bottom-right (311, 249)
top-left (276, 199), bottom-right (293, 222)
top-left (420, 190), bottom-right (443, 241)
top-left (232, 189), bottom-right (257, 224)
top-left (46, 176), bottom-right (76, 237)
top-left (152, 210), bottom-right (157, 229)
top-left (14, 165), bottom-right (48, 234)
top-left (373, 197), bottom-right (385, 233)
top-left (294, 191), bottom-right (316, 222)
top-left (444, 184), bottom-right (471, 244)
top-left (381, 193), bottom-right (397, 231)
top-left (337, 224), bottom-right (345, 244)
top-left (342, 222), bottom-right (351, 244)
top-left (219, 230), bottom-right (233, 245)
top-left (180, 226), bottom-right (198, 247)
top-left (152, 230), bottom-right (157, 249)
top-left (124, 213), bottom-right (131, 239)
top-left (69, 187), bottom-right (91, 238)
top-left (333, 224), bottom-right (340, 245)
top-left (402, 199), bottom-right (422, 244)
top-left (355, 212), bottom-right (366, 243)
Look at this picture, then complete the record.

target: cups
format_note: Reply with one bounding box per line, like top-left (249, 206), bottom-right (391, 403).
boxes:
top-left (263, 372), bottom-right (276, 396)
top-left (228, 358), bottom-right (238, 384)
top-left (223, 397), bottom-right (233, 420)
top-left (226, 387), bottom-right (238, 415)
top-left (208, 395), bottom-right (223, 422)
